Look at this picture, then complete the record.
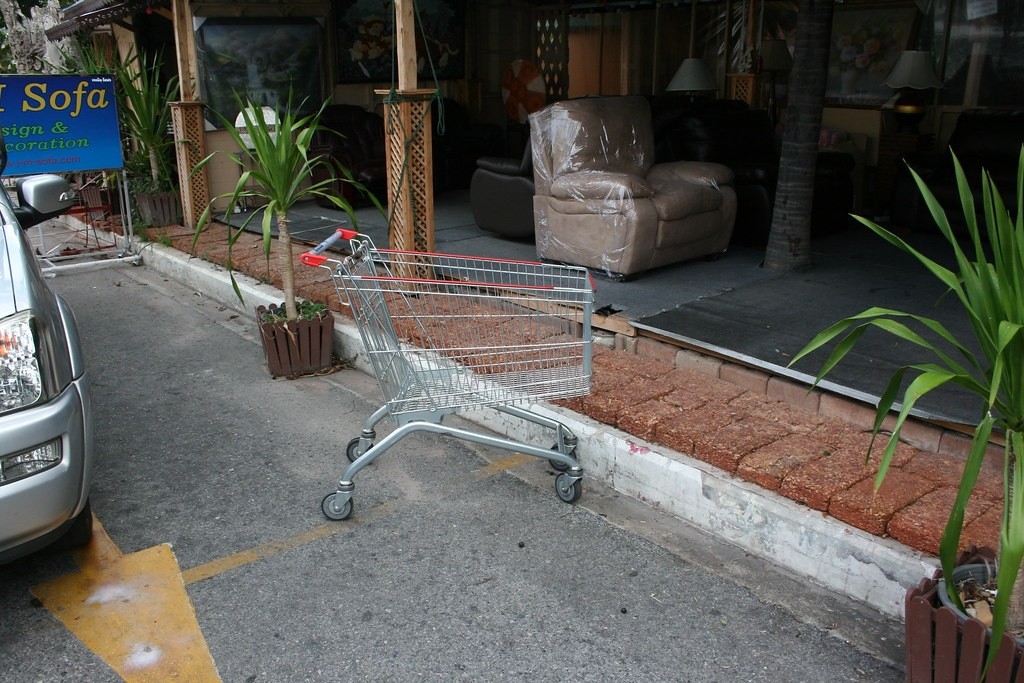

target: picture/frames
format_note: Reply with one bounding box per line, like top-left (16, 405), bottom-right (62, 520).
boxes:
top-left (778, 0), bottom-right (934, 111)
top-left (194, 14), bottom-right (328, 134)
top-left (932, 0), bottom-right (1024, 116)
top-left (329, 0), bottom-right (468, 83)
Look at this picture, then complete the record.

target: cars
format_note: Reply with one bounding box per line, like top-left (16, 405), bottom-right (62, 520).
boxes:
top-left (0, 173), bottom-right (97, 575)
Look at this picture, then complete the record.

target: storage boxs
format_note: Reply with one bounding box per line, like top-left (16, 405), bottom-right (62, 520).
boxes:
top-left (817, 130), bottom-right (869, 225)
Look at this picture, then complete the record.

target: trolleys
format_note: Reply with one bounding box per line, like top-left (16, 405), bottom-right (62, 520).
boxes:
top-left (298, 226), bottom-right (595, 519)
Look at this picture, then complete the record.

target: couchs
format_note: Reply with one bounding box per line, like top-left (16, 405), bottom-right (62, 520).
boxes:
top-left (305, 102), bottom-right (385, 213)
top-left (528, 95), bottom-right (739, 282)
top-left (667, 98), bottom-right (856, 246)
top-left (652, 119), bottom-right (673, 164)
top-left (430, 96), bottom-right (504, 194)
top-left (891, 107), bottom-right (1024, 243)
top-left (469, 133), bottom-right (536, 240)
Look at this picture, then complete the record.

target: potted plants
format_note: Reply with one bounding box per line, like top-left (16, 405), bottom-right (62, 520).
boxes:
top-left (33, 36), bottom-right (139, 215)
top-left (784, 147), bottom-right (1024, 683)
top-left (189, 83), bottom-right (392, 382)
top-left (119, 52), bottom-right (184, 227)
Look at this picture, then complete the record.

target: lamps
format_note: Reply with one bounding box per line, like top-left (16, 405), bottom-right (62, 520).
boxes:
top-left (755, 39), bottom-right (794, 105)
top-left (879, 50), bottom-right (944, 134)
top-left (664, 58), bottom-right (720, 103)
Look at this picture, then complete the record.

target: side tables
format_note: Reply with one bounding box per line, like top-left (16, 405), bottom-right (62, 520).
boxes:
top-left (877, 131), bottom-right (938, 228)
